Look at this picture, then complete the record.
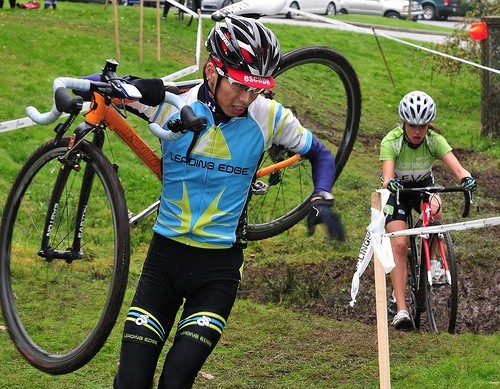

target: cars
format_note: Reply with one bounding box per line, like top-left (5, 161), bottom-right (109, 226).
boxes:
top-left (200, 0), bottom-right (461, 22)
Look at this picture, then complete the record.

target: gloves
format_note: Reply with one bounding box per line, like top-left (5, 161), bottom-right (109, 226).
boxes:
top-left (460, 177), bottom-right (477, 192)
top-left (308, 199), bottom-right (345, 242)
top-left (134, 78), bottom-right (166, 108)
top-left (387, 179), bottom-right (404, 195)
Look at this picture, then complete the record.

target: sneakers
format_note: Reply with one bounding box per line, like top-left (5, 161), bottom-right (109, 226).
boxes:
top-left (391, 310), bottom-right (415, 330)
top-left (431, 269), bottom-right (447, 287)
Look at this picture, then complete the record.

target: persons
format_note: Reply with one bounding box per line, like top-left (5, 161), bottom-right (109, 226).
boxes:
top-left (379, 91), bottom-right (477, 331)
top-left (44, 0), bottom-right (57, 9)
top-left (0, 0), bottom-right (16, 9)
top-left (71, 15), bottom-right (345, 389)
top-left (162, 0), bottom-right (171, 20)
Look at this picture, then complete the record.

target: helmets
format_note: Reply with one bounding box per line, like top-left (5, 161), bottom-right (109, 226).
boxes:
top-left (398, 91), bottom-right (436, 125)
top-left (205, 15), bottom-right (282, 77)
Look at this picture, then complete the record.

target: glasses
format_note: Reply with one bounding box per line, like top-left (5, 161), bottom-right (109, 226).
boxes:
top-left (211, 59), bottom-right (265, 94)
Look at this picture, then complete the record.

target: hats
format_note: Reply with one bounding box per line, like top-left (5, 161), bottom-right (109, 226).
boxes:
top-left (212, 33), bottom-right (275, 89)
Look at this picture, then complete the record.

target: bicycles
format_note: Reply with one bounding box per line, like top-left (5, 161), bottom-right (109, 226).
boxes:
top-left (387, 185), bottom-right (474, 334)
top-left (0, 0), bottom-right (362, 375)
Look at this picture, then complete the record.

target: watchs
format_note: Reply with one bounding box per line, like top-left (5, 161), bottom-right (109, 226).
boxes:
top-left (311, 191), bottom-right (334, 200)
